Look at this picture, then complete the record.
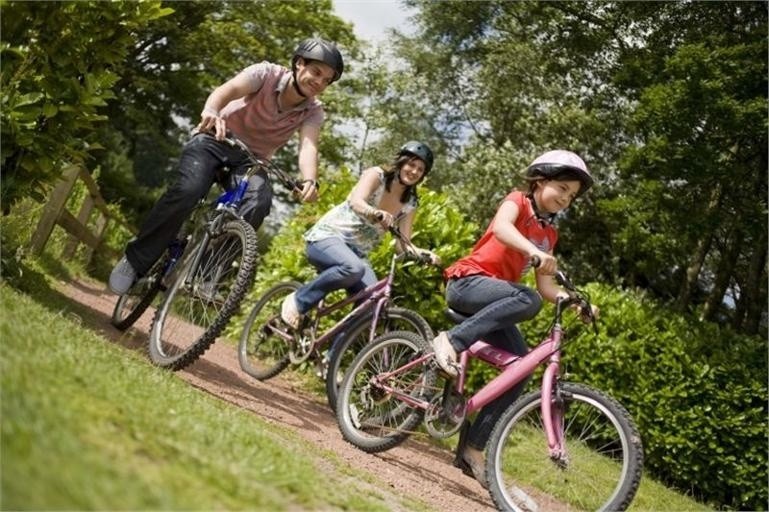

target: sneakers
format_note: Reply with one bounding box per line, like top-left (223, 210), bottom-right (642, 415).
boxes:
top-left (201, 282), bottom-right (224, 302)
top-left (109, 256), bottom-right (138, 298)
top-left (464, 450), bottom-right (487, 486)
top-left (434, 331), bottom-right (461, 378)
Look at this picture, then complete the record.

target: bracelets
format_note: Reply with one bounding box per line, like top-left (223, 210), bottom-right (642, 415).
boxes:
top-left (364, 205), bottom-right (374, 219)
top-left (302, 178), bottom-right (320, 190)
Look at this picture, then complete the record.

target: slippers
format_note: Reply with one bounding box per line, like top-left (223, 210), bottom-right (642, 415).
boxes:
top-left (281, 292), bottom-right (300, 330)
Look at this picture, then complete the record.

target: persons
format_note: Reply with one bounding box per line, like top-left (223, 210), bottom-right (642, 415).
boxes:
top-left (279, 139), bottom-right (444, 388)
top-left (429, 149), bottom-right (594, 493)
top-left (106, 35), bottom-right (345, 298)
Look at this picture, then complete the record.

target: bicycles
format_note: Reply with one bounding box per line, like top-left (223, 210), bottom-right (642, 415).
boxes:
top-left (333, 254), bottom-right (645, 512)
top-left (237, 210), bottom-right (446, 433)
top-left (111, 122), bottom-right (308, 372)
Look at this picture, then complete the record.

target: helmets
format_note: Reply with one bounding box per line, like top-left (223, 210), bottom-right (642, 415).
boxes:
top-left (528, 148), bottom-right (595, 198)
top-left (402, 140), bottom-right (434, 168)
top-left (297, 38), bottom-right (343, 80)
